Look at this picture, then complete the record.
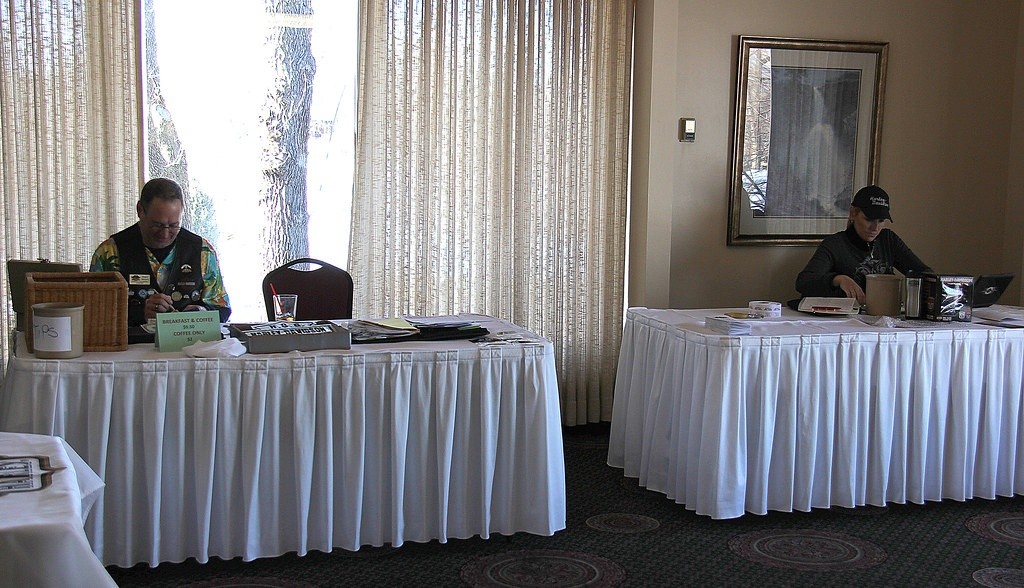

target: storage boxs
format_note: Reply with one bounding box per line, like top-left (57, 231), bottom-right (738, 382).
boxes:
top-left (865, 273), bottom-right (904, 316)
top-left (24, 271), bottom-right (128, 353)
top-left (229, 320), bottom-right (351, 354)
top-left (921, 272), bottom-right (973, 322)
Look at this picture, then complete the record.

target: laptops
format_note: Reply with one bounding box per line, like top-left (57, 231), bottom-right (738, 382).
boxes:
top-left (971, 274), bottom-right (1015, 308)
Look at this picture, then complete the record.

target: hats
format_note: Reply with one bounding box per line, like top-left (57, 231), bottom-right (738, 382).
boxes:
top-left (853, 185), bottom-right (894, 223)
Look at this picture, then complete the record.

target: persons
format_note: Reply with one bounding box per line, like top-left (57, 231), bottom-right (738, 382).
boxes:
top-left (795, 185), bottom-right (936, 307)
top-left (89, 177), bottom-right (232, 326)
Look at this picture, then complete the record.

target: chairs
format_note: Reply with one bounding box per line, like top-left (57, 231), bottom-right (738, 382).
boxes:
top-left (262, 257), bottom-right (354, 322)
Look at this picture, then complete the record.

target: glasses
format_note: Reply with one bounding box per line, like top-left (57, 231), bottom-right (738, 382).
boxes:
top-left (141, 203), bottom-right (181, 233)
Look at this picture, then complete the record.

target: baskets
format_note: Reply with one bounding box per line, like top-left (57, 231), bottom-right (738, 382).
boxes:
top-left (24, 272), bottom-right (130, 352)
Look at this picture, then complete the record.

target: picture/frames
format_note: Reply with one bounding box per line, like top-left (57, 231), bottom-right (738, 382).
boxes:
top-left (726, 34), bottom-right (890, 247)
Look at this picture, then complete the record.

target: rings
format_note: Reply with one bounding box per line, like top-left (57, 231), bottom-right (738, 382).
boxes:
top-left (155, 304), bottom-right (159, 311)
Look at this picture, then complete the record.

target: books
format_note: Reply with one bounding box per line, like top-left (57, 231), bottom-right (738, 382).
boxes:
top-left (345, 317), bottom-right (419, 342)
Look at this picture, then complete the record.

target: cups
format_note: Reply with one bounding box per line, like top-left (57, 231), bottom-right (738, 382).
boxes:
top-left (866, 274), bottom-right (900, 317)
top-left (904, 270), bottom-right (922, 320)
top-left (273, 294), bottom-right (298, 321)
top-left (31, 303), bottom-right (85, 359)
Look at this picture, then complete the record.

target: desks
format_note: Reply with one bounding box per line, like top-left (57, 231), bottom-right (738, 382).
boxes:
top-left (0, 313), bottom-right (568, 570)
top-left (606, 304), bottom-right (1024, 524)
top-left (0, 430), bottom-right (119, 588)
